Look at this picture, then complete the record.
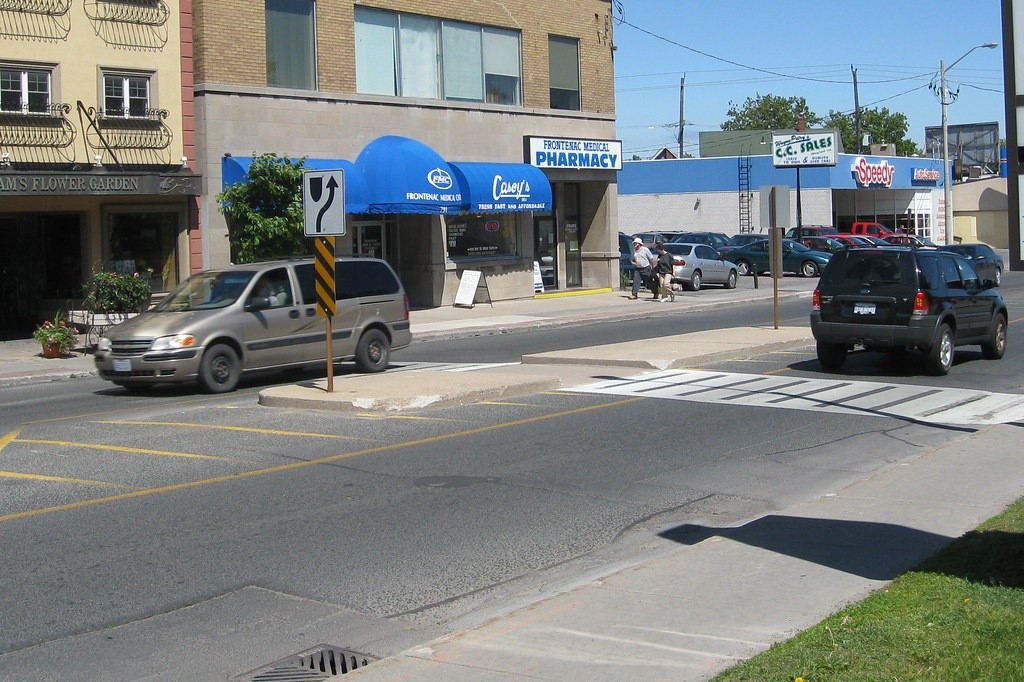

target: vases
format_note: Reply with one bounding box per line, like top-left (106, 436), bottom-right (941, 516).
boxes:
top-left (42, 343), bottom-right (60, 358)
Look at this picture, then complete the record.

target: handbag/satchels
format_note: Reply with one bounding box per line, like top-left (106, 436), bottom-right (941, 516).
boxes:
top-left (672, 276), bottom-right (683, 292)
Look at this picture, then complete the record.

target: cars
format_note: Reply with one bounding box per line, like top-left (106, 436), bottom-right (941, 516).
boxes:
top-left (834, 236), bottom-right (891, 248)
top-left (934, 243), bottom-right (1004, 288)
top-left (804, 235), bottom-right (864, 250)
top-left (790, 235), bottom-right (845, 254)
top-left (881, 234), bottom-right (938, 250)
top-left (716, 233), bottom-right (768, 258)
top-left (726, 238), bottom-right (834, 277)
top-left (650, 243), bottom-right (739, 291)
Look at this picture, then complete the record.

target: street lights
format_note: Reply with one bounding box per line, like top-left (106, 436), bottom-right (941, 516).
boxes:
top-left (939, 42), bottom-right (998, 245)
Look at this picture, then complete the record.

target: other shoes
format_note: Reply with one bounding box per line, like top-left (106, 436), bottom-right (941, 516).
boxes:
top-left (670, 293), bottom-right (675, 302)
top-left (652, 292), bottom-right (659, 298)
top-left (660, 297), bottom-right (668, 302)
top-left (627, 294), bottom-right (638, 299)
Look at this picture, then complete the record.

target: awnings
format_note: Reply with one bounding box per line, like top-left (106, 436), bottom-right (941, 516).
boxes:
top-left (221, 135), bottom-right (553, 215)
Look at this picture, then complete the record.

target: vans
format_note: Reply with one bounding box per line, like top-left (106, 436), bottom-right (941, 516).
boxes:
top-left (95, 252), bottom-right (414, 393)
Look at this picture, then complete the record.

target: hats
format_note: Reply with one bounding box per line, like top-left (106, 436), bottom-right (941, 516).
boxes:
top-left (632, 237), bottom-right (644, 245)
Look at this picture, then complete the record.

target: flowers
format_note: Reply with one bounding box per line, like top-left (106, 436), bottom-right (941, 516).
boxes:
top-left (32, 309), bottom-right (80, 355)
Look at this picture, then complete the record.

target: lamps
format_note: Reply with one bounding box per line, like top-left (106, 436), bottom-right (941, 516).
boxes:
top-left (180, 156), bottom-right (189, 168)
top-left (1, 153), bottom-right (12, 168)
top-left (94, 155), bottom-right (103, 168)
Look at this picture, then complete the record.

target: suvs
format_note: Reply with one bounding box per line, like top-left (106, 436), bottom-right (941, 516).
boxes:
top-left (810, 242), bottom-right (1008, 375)
top-left (632, 230), bottom-right (730, 249)
top-left (785, 226), bottom-right (838, 242)
top-left (619, 232), bottom-right (637, 280)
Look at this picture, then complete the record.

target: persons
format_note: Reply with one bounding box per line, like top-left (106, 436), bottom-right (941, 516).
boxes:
top-left (628, 238), bottom-right (675, 303)
top-left (908, 227), bottom-right (914, 243)
top-left (896, 224), bottom-right (904, 233)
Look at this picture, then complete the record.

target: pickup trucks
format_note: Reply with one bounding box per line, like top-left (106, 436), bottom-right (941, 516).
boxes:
top-left (838, 223), bottom-right (905, 243)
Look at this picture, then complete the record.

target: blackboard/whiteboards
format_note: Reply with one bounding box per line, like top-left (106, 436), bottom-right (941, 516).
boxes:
top-left (454, 270), bottom-right (491, 305)
